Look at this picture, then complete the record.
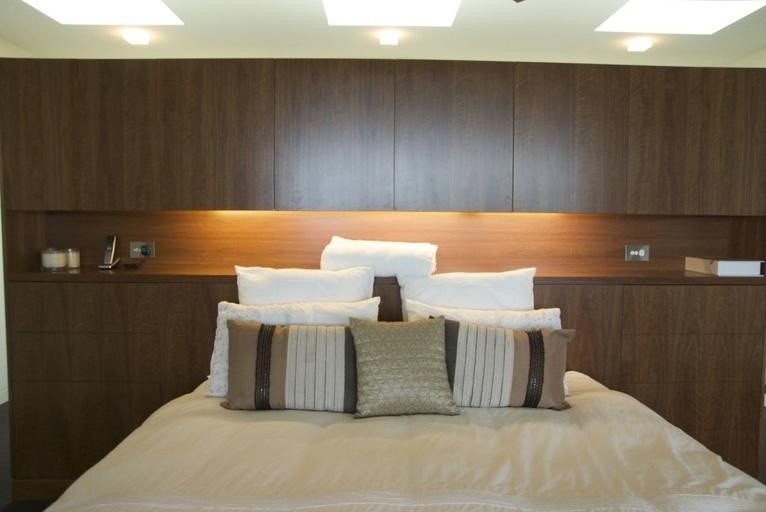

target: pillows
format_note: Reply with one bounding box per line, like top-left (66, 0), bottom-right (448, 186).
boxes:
top-left (319, 235), bottom-right (439, 276)
top-left (344, 312), bottom-right (461, 420)
top-left (403, 297), bottom-right (570, 397)
top-left (426, 315), bottom-right (577, 412)
top-left (233, 263), bottom-right (375, 305)
top-left (217, 317), bottom-right (358, 415)
top-left (395, 265), bottom-right (538, 321)
top-left (203, 296), bottom-right (384, 400)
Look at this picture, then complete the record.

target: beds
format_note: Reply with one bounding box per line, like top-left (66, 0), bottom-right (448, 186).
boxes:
top-left (42, 369), bottom-right (766, 509)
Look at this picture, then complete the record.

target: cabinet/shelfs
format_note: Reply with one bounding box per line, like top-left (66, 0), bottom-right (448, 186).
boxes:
top-left (0, 58), bottom-right (766, 508)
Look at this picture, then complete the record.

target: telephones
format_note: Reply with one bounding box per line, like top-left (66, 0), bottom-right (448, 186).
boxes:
top-left (96, 234), bottom-right (120, 269)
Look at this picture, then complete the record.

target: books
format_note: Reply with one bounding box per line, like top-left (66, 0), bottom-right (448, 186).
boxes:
top-left (683, 254), bottom-right (766, 278)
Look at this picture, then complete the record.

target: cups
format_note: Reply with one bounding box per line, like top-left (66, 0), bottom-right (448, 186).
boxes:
top-left (41, 247), bottom-right (66, 270)
top-left (65, 248), bottom-right (81, 269)
